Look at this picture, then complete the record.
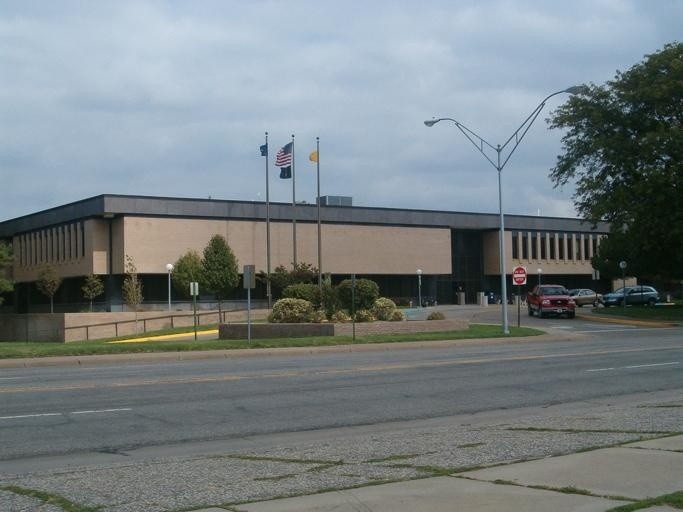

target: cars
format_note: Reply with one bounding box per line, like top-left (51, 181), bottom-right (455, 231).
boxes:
top-left (604, 285), bottom-right (659, 307)
top-left (569, 288), bottom-right (603, 306)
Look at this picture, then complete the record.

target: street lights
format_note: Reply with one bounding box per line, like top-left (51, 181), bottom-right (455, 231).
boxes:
top-left (425, 85), bottom-right (586, 331)
top-left (167, 264), bottom-right (174, 309)
top-left (537, 268), bottom-right (542, 285)
top-left (416, 268), bottom-right (423, 306)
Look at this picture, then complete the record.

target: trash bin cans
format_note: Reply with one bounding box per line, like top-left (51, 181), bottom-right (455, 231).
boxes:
top-left (482, 296), bottom-right (488, 307)
top-left (515, 295), bottom-right (521, 305)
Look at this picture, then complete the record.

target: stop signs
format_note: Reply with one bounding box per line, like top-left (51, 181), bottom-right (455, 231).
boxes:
top-left (512, 266), bottom-right (527, 284)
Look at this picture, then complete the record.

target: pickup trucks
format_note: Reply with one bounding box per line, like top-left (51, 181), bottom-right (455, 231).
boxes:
top-left (529, 285), bottom-right (576, 317)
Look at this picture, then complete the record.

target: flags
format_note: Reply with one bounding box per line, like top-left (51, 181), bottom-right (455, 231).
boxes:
top-left (309, 151), bottom-right (318, 162)
top-left (275, 142), bottom-right (292, 179)
top-left (260, 144), bottom-right (268, 156)
